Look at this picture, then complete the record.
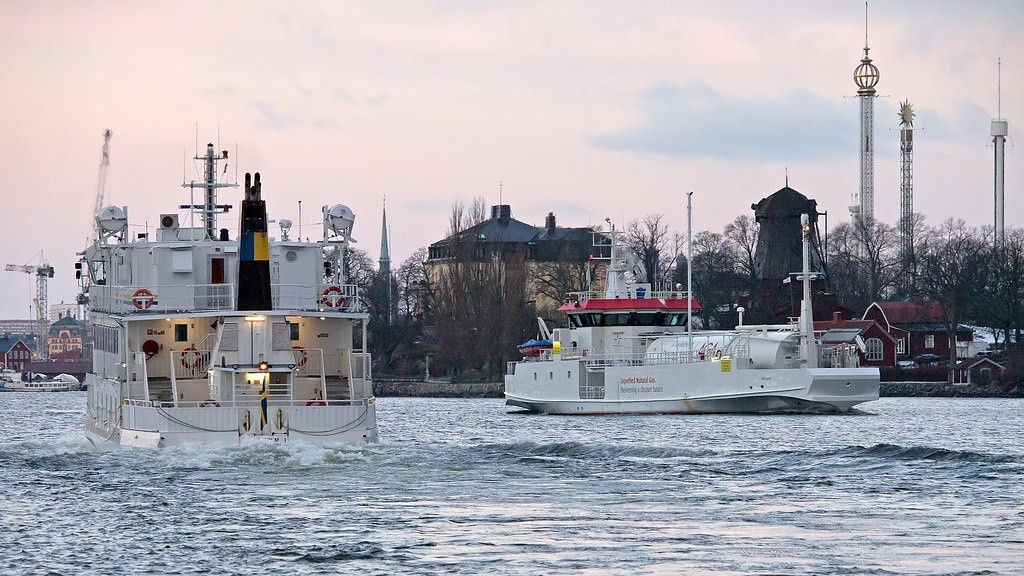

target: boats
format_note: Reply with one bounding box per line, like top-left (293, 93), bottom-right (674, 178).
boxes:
top-left (504, 191), bottom-right (879, 414)
top-left (0, 367), bottom-right (71, 392)
top-left (75, 121), bottom-right (378, 449)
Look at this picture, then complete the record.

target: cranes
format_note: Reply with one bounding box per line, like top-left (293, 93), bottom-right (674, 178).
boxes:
top-left (5, 250), bottom-right (55, 362)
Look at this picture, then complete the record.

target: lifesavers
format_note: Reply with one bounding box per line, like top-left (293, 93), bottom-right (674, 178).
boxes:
top-left (293, 345), bottom-right (307, 367)
top-left (132, 288), bottom-right (152, 309)
top-left (200, 400), bottom-right (221, 407)
top-left (180, 348), bottom-right (201, 368)
top-left (306, 397), bottom-right (326, 407)
top-left (323, 286), bottom-right (345, 307)
